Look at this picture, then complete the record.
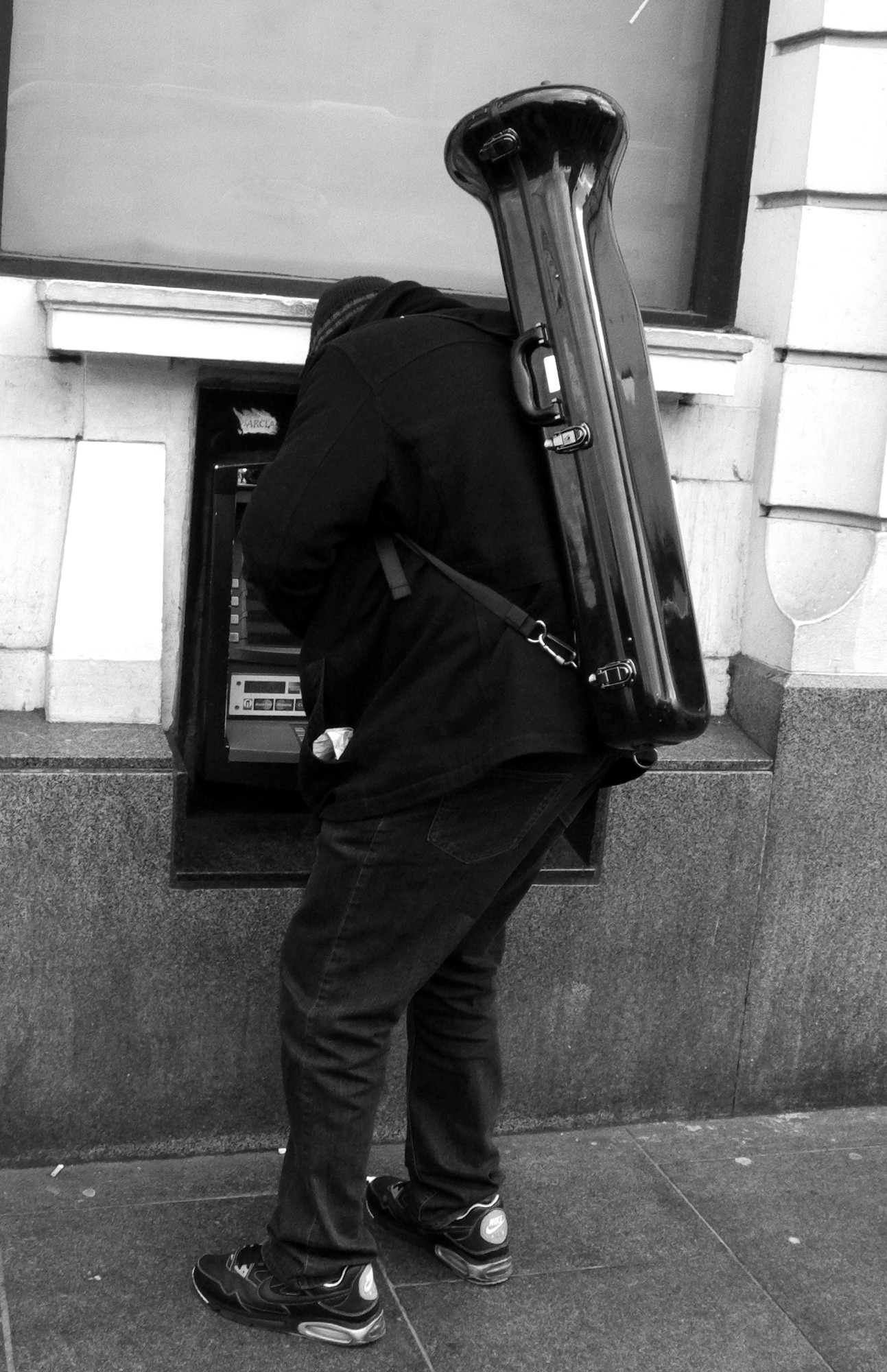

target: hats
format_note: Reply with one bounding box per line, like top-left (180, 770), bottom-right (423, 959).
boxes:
top-left (307, 276), bottom-right (392, 356)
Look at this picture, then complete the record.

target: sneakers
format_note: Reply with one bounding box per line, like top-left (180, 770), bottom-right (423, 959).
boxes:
top-left (366, 1172), bottom-right (513, 1287)
top-left (189, 1233), bottom-right (389, 1346)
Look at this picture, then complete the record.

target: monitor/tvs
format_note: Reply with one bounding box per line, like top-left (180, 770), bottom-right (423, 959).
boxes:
top-left (229, 490), bottom-right (307, 666)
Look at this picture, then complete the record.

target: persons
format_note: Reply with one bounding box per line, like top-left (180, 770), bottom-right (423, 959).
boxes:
top-left (189, 277), bottom-right (658, 1351)
